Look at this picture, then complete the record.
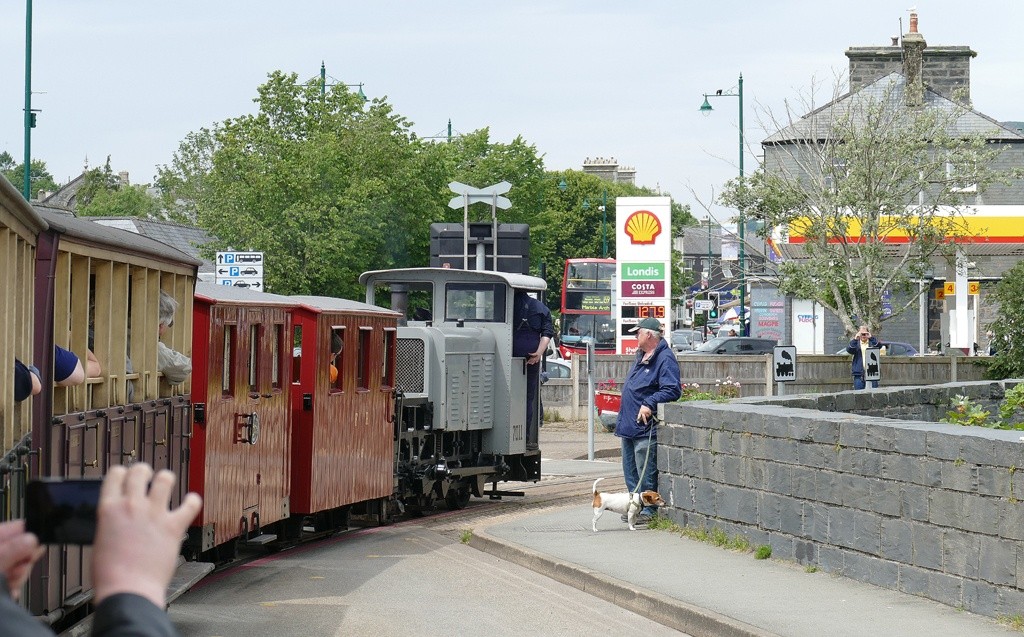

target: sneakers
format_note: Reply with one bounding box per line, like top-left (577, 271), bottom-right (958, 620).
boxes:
top-left (621, 512), bottom-right (655, 523)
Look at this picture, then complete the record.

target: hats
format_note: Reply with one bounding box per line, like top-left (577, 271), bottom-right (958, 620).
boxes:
top-left (858, 326), bottom-right (869, 334)
top-left (628, 317), bottom-right (663, 333)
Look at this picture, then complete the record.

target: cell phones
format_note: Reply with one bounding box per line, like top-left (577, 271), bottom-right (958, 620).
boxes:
top-left (23, 477), bottom-right (103, 547)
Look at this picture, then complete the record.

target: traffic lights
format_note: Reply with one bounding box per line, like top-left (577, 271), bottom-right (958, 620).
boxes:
top-left (708, 292), bottom-right (720, 319)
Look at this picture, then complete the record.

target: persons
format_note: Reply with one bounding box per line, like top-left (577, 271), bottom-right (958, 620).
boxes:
top-left (986, 330), bottom-right (998, 356)
top-left (330, 333), bottom-right (344, 384)
top-left (14, 288), bottom-right (191, 402)
top-left (846, 326), bottom-right (883, 390)
top-left (513, 289), bottom-right (554, 451)
top-left (0, 460), bottom-right (205, 637)
top-left (613, 318), bottom-right (681, 525)
top-left (728, 329), bottom-right (737, 337)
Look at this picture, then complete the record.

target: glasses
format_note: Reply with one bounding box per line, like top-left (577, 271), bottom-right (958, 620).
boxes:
top-left (335, 353), bottom-right (340, 359)
top-left (168, 321), bottom-right (173, 328)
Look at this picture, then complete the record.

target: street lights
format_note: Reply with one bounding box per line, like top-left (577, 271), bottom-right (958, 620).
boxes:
top-left (701, 93), bottom-right (745, 336)
top-left (697, 224), bottom-right (711, 289)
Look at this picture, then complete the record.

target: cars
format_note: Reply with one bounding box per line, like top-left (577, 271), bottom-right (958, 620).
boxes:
top-left (671, 333), bottom-right (691, 352)
top-left (676, 324), bottom-right (740, 350)
top-left (835, 342), bottom-right (919, 354)
top-left (543, 359), bottom-right (572, 378)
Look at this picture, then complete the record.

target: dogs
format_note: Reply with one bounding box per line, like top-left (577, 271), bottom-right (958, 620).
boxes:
top-left (592, 478), bottom-right (667, 532)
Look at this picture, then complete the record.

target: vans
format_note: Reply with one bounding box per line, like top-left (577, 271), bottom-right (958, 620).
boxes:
top-left (693, 336), bottom-right (779, 355)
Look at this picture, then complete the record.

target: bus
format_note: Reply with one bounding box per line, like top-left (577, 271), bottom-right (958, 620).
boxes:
top-left (1, 173), bottom-right (545, 636)
top-left (556, 257), bottom-right (616, 358)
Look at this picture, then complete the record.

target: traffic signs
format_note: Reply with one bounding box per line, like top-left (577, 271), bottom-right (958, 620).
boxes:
top-left (215, 253), bottom-right (265, 293)
